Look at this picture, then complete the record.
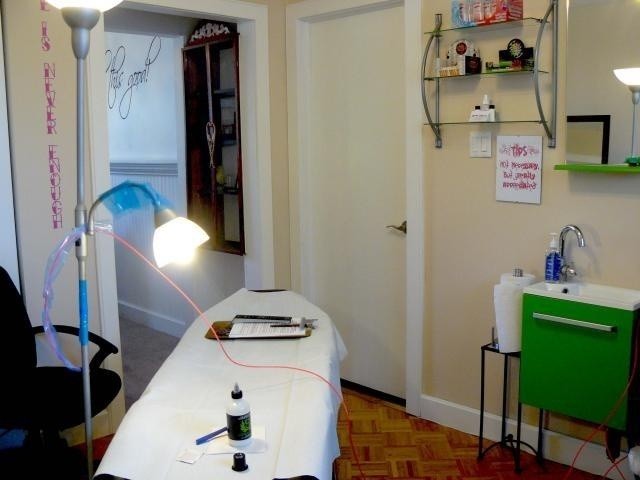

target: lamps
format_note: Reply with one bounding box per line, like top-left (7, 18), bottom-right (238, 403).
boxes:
top-left (610, 66), bottom-right (638, 165)
top-left (42, 1), bottom-right (212, 480)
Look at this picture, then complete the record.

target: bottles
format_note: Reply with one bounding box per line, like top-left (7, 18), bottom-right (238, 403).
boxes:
top-left (225, 382), bottom-right (251, 448)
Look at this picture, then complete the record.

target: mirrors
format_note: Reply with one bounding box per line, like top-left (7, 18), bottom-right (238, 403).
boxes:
top-left (565, 2), bottom-right (639, 168)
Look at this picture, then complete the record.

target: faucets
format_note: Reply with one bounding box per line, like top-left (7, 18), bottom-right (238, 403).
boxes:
top-left (559, 224), bottom-right (585, 282)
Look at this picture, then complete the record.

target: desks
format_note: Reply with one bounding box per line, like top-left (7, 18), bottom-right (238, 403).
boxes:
top-left (92, 288), bottom-right (346, 480)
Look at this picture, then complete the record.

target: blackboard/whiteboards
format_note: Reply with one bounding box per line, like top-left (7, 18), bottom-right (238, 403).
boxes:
top-left (102, 31), bottom-right (179, 177)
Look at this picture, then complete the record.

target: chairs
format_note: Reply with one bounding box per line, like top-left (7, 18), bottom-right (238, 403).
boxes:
top-left (0, 264), bottom-right (120, 480)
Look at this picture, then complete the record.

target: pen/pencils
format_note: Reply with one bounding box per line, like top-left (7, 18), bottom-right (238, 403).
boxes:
top-left (270, 324), bottom-right (301, 327)
top-left (195, 427), bottom-right (227, 445)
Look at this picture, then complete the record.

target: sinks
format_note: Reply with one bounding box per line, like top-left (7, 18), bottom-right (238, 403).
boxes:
top-left (547, 280), bottom-right (640, 311)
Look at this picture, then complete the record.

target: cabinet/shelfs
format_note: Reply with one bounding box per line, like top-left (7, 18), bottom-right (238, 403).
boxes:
top-left (478, 338), bottom-right (543, 474)
top-left (183, 20), bottom-right (244, 258)
top-left (422, 0), bottom-right (558, 148)
top-left (519, 293), bottom-right (640, 432)
